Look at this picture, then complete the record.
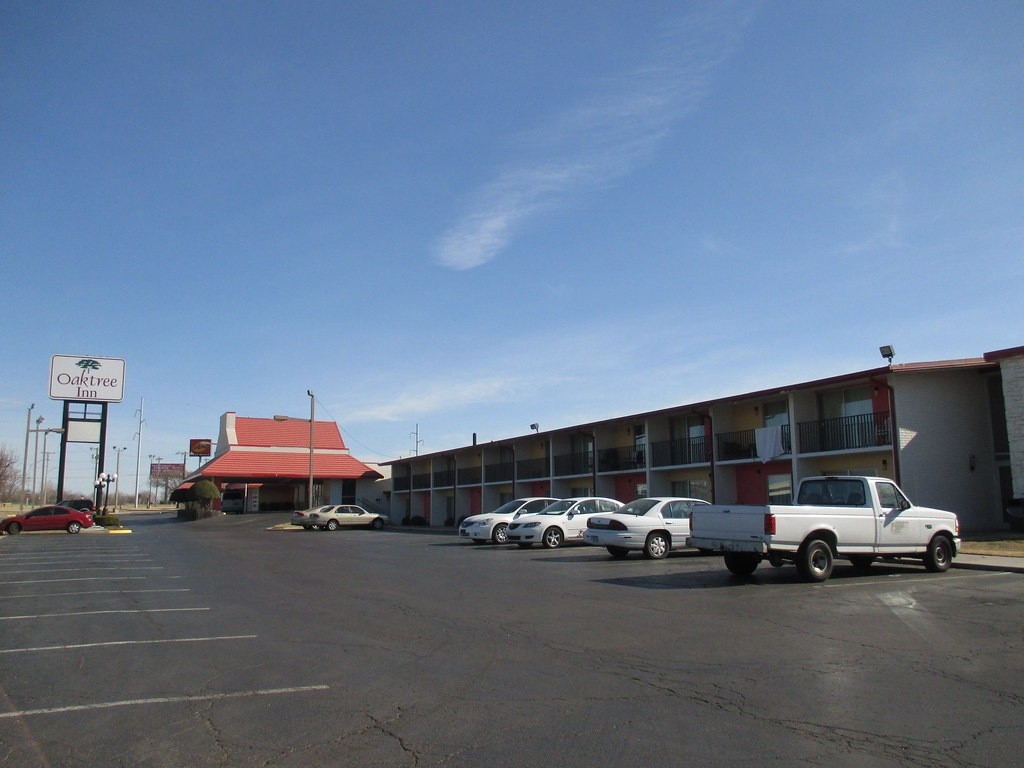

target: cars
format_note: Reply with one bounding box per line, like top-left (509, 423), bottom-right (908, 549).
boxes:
top-left (583, 496), bottom-right (714, 561)
top-left (308, 505), bottom-right (389, 531)
top-left (505, 496), bottom-right (625, 549)
top-left (0, 505), bottom-right (94, 535)
top-left (458, 498), bottom-right (564, 545)
top-left (290, 505), bottom-right (334, 531)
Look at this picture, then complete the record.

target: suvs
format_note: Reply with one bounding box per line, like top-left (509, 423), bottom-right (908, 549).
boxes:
top-left (56, 499), bottom-right (96, 512)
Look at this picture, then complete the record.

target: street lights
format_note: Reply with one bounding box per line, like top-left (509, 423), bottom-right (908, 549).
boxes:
top-left (95, 478), bottom-right (105, 516)
top-left (31, 415), bottom-right (45, 510)
top-left (113, 446), bottom-right (127, 513)
top-left (19, 403), bottom-right (34, 516)
top-left (176, 452), bottom-right (194, 480)
top-left (307, 390), bottom-right (315, 509)
top-left (100, 473), bottom-right (117, 517)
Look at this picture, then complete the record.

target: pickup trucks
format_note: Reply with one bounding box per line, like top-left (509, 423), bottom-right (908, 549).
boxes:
top-left (685, 474), bottom-right (961, 584)
